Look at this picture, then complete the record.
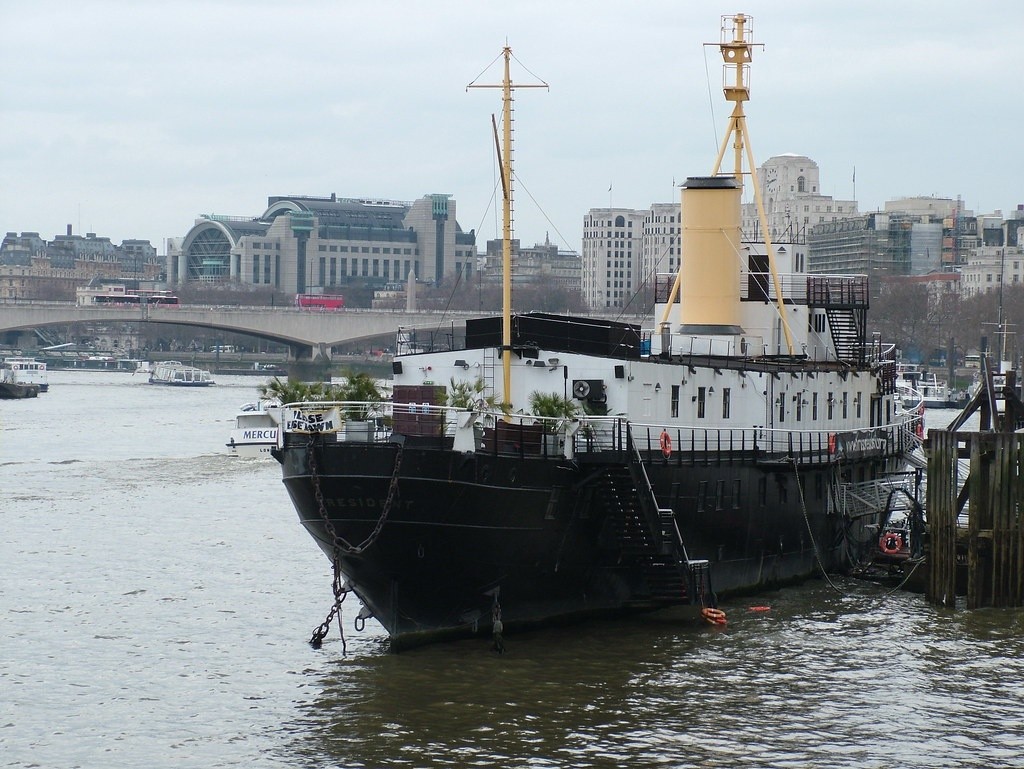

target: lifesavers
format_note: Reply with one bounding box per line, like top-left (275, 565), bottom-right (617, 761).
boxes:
top-left (917, 425), bottom-right (924, 440)
top-left (748, 605), bottom-right (770, 613)
top-left (827, 433), bottom-right (837, 454)
top-left (881, 533), bottom-right (903, 555)
top-left (700, 609), bottom-right (726, 625)
top-left (920, 402), bottom-right (924, 415)
top-left (660, 431), bottom-right (673, 454)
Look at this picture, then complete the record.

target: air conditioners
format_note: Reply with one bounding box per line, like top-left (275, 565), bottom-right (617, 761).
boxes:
top-left (573, 379), bottom-right (604, 399)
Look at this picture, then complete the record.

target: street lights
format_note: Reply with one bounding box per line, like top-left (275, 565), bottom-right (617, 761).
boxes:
top-left (132, 249), bottom-right (138, 291)
top-left (309, 257), bottom-right (314, 295)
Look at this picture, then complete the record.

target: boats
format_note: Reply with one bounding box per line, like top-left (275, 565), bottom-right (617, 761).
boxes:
top-left (0, 354), bottom-right (50, 399)
top-left (148, 360), bottom-right (217, 387)
top-left (267, 11), bottom-right (1022, 655)
top-left (225, 392), bottom-right (396, 466)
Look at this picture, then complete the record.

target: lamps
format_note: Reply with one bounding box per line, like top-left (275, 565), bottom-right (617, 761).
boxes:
top-left (532, 361), bottom-right (546, 367)
top-left (454, 360), bottom-right (470, 370)
top-left (853, 398), bottom-right (858, 405)
top-left (840, 398), bottom-right (848, 406)
top-left (776, 398), bottom-right (781, 406)
top-left (827, 398), bottom-right (835, 403)
top-left (548, 358), bottom-right (559, 364)
top-left (803, 400), bottom-right (808, 407)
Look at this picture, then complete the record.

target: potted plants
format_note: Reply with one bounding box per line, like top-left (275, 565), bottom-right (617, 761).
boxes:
top-left (335, 368), bottom-right (380, 442)
top-left (447, 378), bottom-right (512, 449)
top-left (528, 391), bottom-right (580, 454)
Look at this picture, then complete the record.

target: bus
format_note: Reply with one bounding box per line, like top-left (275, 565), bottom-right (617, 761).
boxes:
top-left (210, 345), bottom-right (235, 354)
top-left (92, 293), bottom-right (180, 311)
top-left (294, 292), bottom-right (345, 309)
top-left (126, 289), bottom-right (172, 299)
